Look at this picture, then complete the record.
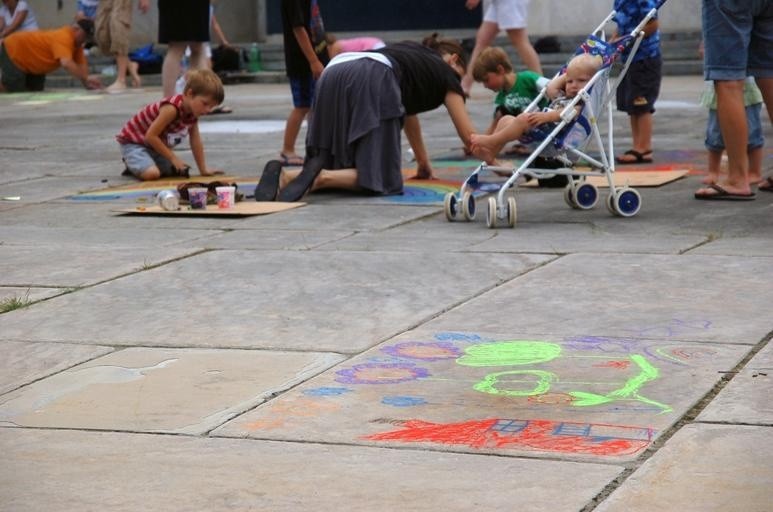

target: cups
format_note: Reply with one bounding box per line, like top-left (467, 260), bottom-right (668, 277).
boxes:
top-left (155, 184), bottom-right (237, 211)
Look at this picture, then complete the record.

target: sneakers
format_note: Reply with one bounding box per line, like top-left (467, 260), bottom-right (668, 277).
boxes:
top-left (254, 159), bottom-right (280, 201)
top-left (281, 156), bottom-right (325, 201)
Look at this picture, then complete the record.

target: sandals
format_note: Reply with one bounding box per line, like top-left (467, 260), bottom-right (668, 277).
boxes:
top-left (755, 176), bottom-right (773, 194)
top-left (614, 147), bottom-right (655, 164)
top-left (277, 151), bottom-right (306, 167)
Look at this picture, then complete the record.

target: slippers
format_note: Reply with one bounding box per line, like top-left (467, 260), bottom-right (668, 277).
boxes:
top-left (212, 105), bottom-right (233, 114)
top-left (695, 184), bottom-right (758, 202)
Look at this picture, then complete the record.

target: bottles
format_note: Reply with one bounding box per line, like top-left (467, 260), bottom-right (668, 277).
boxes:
top-left (247, 41), bottom-right (262, 72)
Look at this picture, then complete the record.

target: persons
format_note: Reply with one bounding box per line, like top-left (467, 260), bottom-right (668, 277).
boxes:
top-left (694, 1), bottom-right (770, 201)
top-left (472, 45), bottom-right (552, 158)
top-left (323, 28), bottom-right (390, 61)
top-left (277, 3), bottom-right (331, 164)
top-left (463, 54), bottom-right (604, 166)
top-left (252, 32), bottom-right (515, 202)
top-left (117, 68), bottom-right (224, 180)
top-left (457, 0), bottom-right (547, 98)
top-left (0, 1), bottom-right (238, 116)
top-left (699, 37), bottom-right (762, 186)
top-left (609, 1), bottom-right (662, 164)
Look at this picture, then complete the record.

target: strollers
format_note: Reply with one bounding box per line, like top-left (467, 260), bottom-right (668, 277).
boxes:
top-left (436, 0), bottom-right (667, 232)
